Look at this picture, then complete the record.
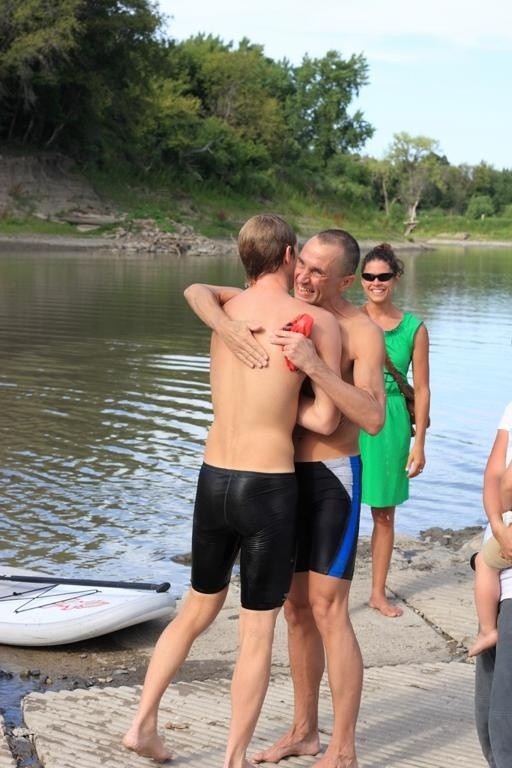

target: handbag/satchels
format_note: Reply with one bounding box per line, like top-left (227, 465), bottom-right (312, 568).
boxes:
top-left (404, 386), bottom-right (431, 429)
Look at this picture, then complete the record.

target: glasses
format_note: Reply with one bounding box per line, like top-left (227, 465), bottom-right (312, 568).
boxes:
top-left (359, 273), bottom-right (394, 281)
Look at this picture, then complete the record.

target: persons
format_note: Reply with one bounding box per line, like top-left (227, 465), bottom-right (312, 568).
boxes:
top-left (466, 461), bottom-right (511, 656)
top-left (474, 397), bottom-right (512, 767)
top-left (118, 214), bottom-right (342, 767)
top-left (183, 228), bottom-right (388, 768)
top-left (355, 241), bottom-right (430, 621)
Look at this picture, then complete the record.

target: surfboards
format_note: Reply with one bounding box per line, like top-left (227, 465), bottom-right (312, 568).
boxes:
top-left (0, 566), bottom-right (177, 648)
